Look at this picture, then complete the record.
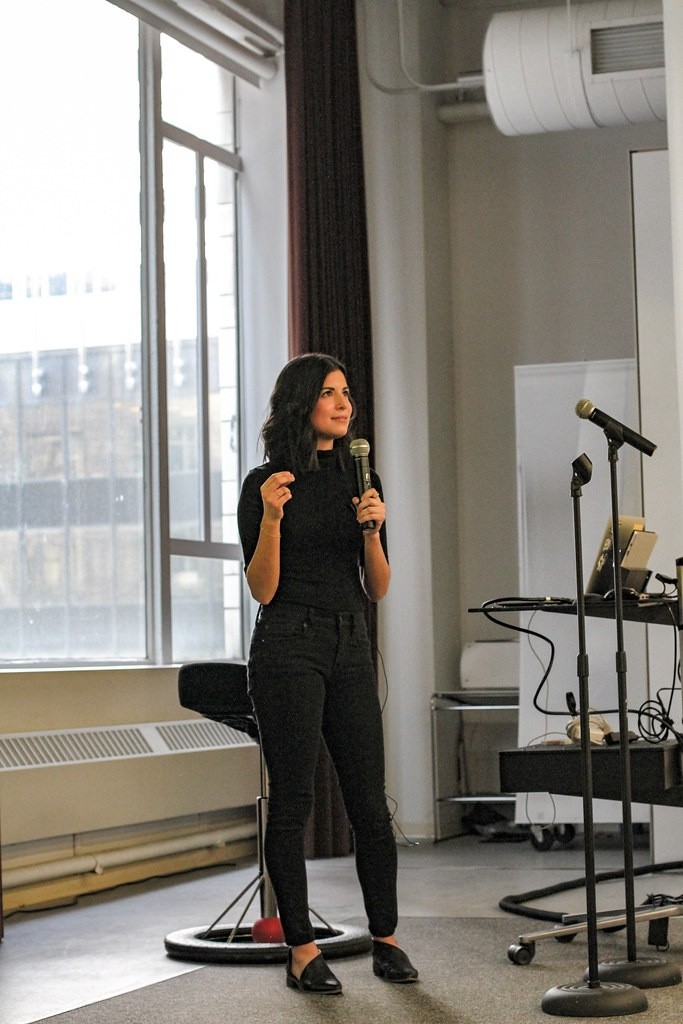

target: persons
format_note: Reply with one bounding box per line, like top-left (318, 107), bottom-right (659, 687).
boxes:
top-left (239, 352), bottom-right (420, 998)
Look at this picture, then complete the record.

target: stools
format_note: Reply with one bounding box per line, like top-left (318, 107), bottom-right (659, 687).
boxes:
top-left (164, 663), bottom-right (368, 959)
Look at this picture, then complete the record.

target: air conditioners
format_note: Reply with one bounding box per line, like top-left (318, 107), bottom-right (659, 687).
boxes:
top-left (481, 0), bottom-right (667, 138)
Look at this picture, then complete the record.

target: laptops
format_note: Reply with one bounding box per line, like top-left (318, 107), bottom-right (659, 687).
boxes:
top-left (496, 515), bottom-right (647, 606)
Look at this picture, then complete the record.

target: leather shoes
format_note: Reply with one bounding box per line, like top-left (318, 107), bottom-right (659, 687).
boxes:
top-left (283, 948), bottom-right (344, 996)
top-left (369, 938), bottom-right (419, 984)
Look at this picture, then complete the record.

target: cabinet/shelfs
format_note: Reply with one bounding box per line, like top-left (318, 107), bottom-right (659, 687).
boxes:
top-left (432, 687), bottom-right (520, 844)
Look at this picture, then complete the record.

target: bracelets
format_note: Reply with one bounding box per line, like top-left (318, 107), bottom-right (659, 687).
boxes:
top-left (259, 526), bottom-right (279, 539)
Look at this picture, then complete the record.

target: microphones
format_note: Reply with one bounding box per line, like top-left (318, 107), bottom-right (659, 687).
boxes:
top-left (350, 439), bottom-right (377, 531)
top-left (575, 399), bottom-right (657, 457)
